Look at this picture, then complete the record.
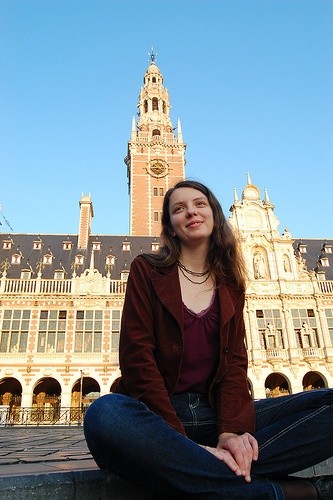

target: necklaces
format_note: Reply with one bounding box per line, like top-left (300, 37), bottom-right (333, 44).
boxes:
top-left (178, 259), bottom-right (212, 284)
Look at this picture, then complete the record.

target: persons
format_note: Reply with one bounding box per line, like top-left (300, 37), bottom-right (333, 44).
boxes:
top-left (83, 180), bottom-right (333, 500)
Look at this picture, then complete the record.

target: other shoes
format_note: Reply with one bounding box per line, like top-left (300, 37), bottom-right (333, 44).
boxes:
top-left (279, 474), bottom-right (333, 500)
